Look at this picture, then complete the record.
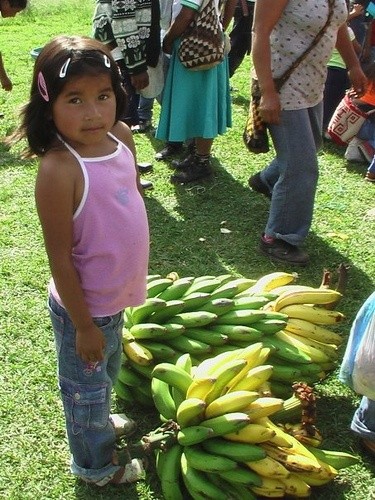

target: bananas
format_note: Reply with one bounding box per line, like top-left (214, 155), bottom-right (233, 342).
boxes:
top-left (153, 341), bottom-right (361, 500)
top-left (112, 272), bottom-right (347, 407)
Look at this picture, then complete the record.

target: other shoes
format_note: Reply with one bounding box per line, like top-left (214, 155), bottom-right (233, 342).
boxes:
top-left (139, 179), bottom-right (153, 190)
top-left (131, 120), bottom-right (150, 132)
top-left (365, 172), bottom-right (375, 181)
top-left (136, 161), bottom-right (152, 173)
top-left (155, 144), bottom-right (183, 159)
top-left (258, 230), bottom-right (309, 266)
top-left (171, 159), bottom-right (213, 183)
top-left (97, 463), bottom-right (143, 486)
top-left (109, 412), bottom-right (139, 438)
top-left (172, 147), bottom-right (195, 168)
top-left (248, 172), bottom-right (272, 201)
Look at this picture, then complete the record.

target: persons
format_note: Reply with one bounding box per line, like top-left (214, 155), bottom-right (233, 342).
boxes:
top-left (0, 0), bottom-right (28, 91)
top-left (3, 36), bottom-right (149, 488)
top-left (322, 0), bottom-right (375, 182)
top-left (248, 0), bottom-right (368, 265)
top-left (93, 0), bottom-right (256, 191)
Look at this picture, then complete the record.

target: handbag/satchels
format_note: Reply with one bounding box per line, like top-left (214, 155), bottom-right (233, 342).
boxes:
top-left (242, 75), bottom-right (268, 153)
top-left (179, 0), bottom-right (224, 71)
top-left (328, 94), bottom-right (375, 143)
